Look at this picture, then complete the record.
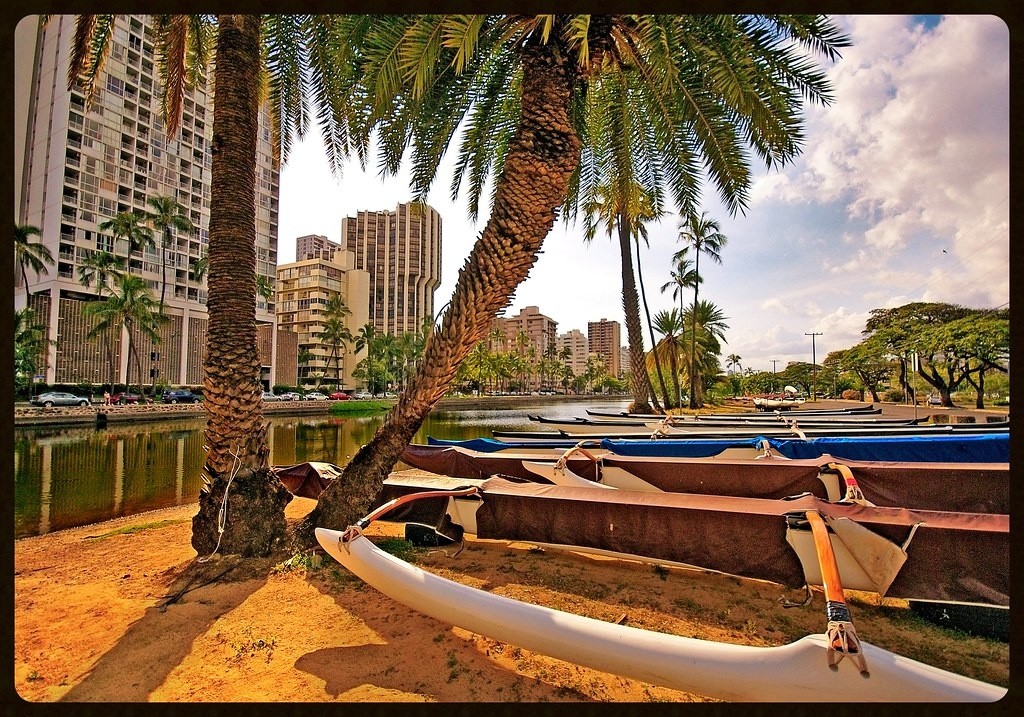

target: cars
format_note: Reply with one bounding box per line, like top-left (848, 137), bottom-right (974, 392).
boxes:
top-left (929, 396), bottom-right (942, 404)
top-left (30, 391), bottom-right (89, 408)
top-left (161, 390), bottom-right (201, 404)
top-left (107, 392), bottom-right (153, 406)
top-left (305, 392), bottom-right (329, 401)
top-left (484, 390), bottom-right (609, 398)
top-left (280, 392), bottom-right (308, 402)
top-left (261, 393), bottom-right (283, 402)
top-left (327, 392), bottom-right (352, 400)
top-left (377, 392), bottom-right (398, 400)
top-left (354, 392), bottom-right (376, 400)
